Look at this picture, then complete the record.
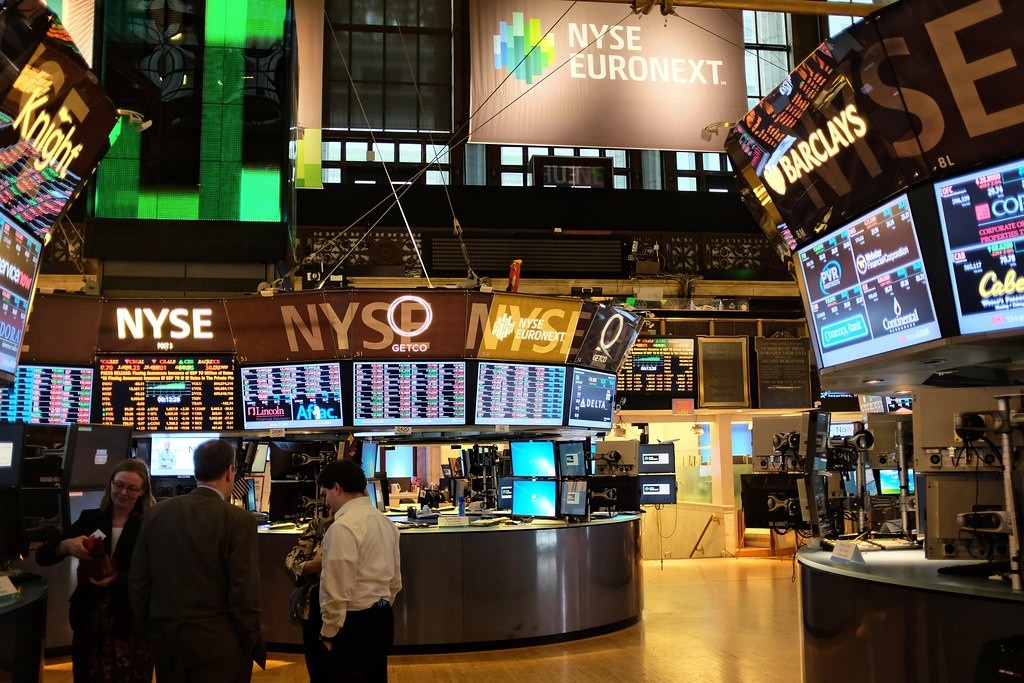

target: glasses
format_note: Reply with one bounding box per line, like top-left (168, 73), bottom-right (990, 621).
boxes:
top-left (232, 466), bottom-right (238, 475)
top-left (110, 477), bottom-right (143, 494)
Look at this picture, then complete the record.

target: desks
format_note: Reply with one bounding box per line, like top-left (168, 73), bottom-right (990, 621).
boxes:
top-left (388, 492), bottom-right (417, 506)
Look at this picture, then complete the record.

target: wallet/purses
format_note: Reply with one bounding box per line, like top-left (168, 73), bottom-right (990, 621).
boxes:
top-left (77, 537), bottom-right (116, 587)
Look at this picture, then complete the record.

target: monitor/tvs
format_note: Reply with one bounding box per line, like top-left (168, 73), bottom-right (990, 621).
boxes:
top-left (596, 440), bottom-right (639, 475)
top-left (60, 422), bottom-right (269, 529)
top-left (237, 358), bottom-right (343, 430)
top-left (94, 351), bottom-right (236, 432)
top-left (933, 159), bottom-right (1024, 334)
top-left (352, 362), bottom-right (467, 426)
top-left (588, 475), bottom-right (640, 513)
top-left (0, 419), bottom-right (29, 560)
top-left (560, 479), bottom-right (589, 522)
top-left (0, 206), bottom-right (44, 383)
top-left (509, 440), bottom-right (559, 478)
top-left (557, 440), bottom-right (588, 478)
top-left (866, 386), bottom-right (1024, 560)
top-left (796, 185), bottom-right (943, 367)
top-left (638, 444), bottom-right (675, 473)
top-left (474, 361), bottom-right (617, 427)
top-left (0, 363), bottom-right (95, 425)
top-left (639, 475), bottom-right (677, 504)
top-left (511, 478), bottom-right (559, 518)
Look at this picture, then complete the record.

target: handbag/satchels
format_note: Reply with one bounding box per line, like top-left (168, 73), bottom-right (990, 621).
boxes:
top-left (288, 578), bottom-right (306, 625)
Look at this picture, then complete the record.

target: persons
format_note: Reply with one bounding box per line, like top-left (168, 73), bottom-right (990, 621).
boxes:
top-left (137, 439), bottom-right (266, 683)
top-left (35, 457), bottom-right (157, 683)
top-left (285, 458), bottom-right (402, 683)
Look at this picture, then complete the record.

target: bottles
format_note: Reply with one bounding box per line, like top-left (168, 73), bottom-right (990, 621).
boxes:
top-left (422, 504), bottom-right (429, 515)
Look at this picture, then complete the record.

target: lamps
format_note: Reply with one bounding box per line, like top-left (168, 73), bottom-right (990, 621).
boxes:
top-left (118, 109), bottom-right (153, 132)
top-left (613, 416), bottom-right (626, 437)
top-left (701, 121), bottom-right (736, 142)
top-left (691, 415), bottom-right (703, 435)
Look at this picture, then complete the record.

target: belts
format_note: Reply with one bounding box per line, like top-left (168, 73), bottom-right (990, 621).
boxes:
top-left (370, 599), bottom-right (388, 609)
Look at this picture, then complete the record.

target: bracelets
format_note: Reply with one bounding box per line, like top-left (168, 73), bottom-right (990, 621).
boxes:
top-left (319, 635), bottom-right (333, 642)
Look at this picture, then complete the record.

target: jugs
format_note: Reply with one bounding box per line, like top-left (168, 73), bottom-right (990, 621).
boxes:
top-left (390, 483), bottom-right (401, 494)
